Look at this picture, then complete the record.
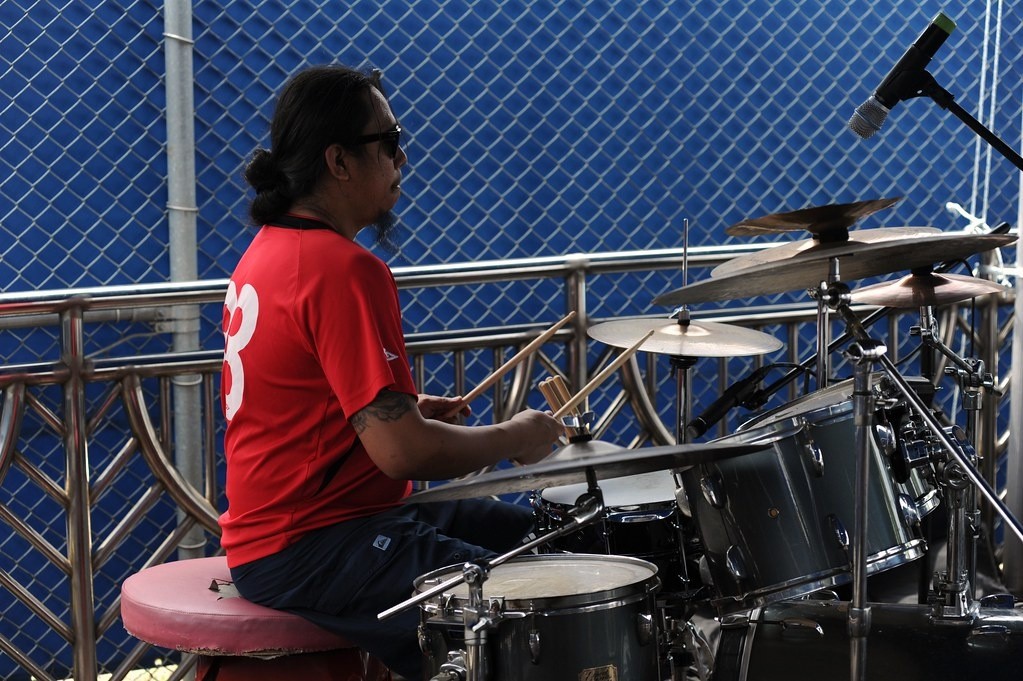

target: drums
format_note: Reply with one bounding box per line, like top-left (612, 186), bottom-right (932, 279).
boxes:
top-left (733, 365), bottom-right (944, 521)
top-left (684, 397), bottom-right (934, 620)
top-left (533, 468), bottom-right (699, 560)
top-left (409, 550), bottom-right (669, 681)
top-left (706, 596), bottom-right (1023, 681)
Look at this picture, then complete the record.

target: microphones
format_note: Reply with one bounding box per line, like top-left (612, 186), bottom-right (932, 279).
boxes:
top-left (687, 366), bottom-right (774, 440)
top-left (848, 12), bottom-right (956, 139)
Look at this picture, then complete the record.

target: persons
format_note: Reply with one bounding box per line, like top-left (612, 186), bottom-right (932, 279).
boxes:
top-left (217, 64), bottom-right (567, 681)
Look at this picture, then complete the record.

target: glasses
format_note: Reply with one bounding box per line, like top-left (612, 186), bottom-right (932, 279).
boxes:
top-left (355, 120), bottom-right (402, 158)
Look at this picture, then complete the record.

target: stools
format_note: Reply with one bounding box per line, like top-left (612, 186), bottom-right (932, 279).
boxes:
top-left (106, 556), bottom-right (363, 681)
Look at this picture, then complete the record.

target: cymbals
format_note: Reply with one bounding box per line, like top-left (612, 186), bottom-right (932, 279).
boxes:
top-left (648, 195), bottom-right (1019, 308)
top-left (401, 438), bottom-right (779, 509)
top-left (708, 194), bottom-right (945, 282)
top-left (586, 315), bottom-right (785, 359)
top-left (837, 270), bottom-right (1011, 309)
